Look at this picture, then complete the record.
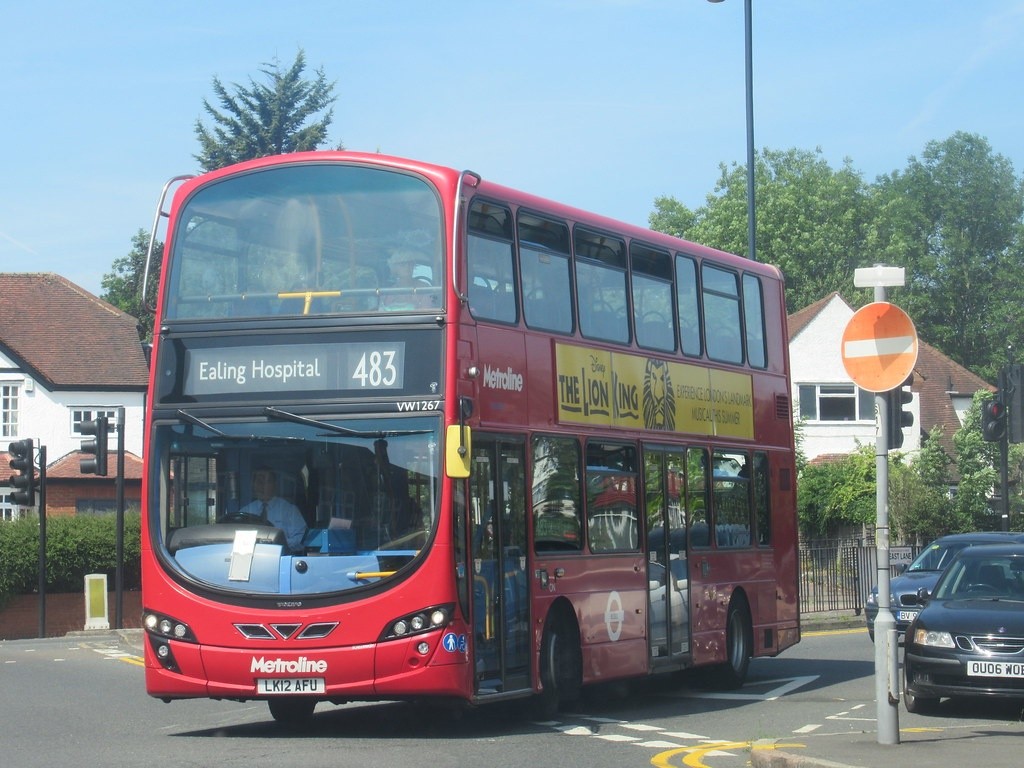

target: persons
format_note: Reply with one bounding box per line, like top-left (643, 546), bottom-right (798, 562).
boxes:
top-left (233, 465), bottom-right (309, 553)
top-left (381, 250), bottom-right (432, 310)
top-left (329, 464), bottom-right (397, 538)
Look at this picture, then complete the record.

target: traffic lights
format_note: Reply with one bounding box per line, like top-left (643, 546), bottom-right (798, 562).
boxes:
top-left (982, 399), bottom-right (1006, 442)
top-left (80, 417), bottom-right (108, 476)
top-left (891, 372), bottom-right (914, 450)
top-left (9, 438), bottom-right (35, 506)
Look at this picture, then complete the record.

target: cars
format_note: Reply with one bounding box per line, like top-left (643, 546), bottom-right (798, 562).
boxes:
top-left (864, 532), bottom-right (1024, 643)
top-left (900, 544), bottom-right (1024, 713)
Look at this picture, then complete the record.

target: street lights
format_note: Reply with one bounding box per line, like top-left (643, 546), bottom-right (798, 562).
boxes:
top-left (853, 264), bottom-right (905, 745)
top-left (710, 0), bottom-right (756, 263)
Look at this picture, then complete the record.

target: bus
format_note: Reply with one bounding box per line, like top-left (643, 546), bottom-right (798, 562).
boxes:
top-left (138, 152), bottom-right (802, 726)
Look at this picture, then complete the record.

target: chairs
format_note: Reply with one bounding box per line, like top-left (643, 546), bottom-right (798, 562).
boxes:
top-left (231, 262), bottom-right (764, 365)
top-left (976, 564), bottom-right (1009, 598)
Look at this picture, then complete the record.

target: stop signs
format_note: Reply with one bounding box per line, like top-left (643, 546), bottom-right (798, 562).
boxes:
top-left (840, 302), bottom-right (919, 393)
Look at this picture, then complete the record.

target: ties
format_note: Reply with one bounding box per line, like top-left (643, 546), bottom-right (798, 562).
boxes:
top-left (260, 503), bottom-right (268, 519)
